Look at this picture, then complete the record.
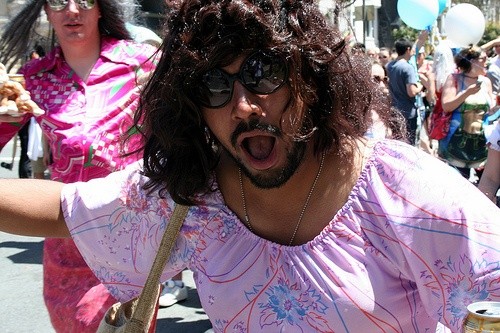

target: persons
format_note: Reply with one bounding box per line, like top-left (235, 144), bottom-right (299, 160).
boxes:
top-left (0, 0), bottom-right (500, 333)
top-left (0, 1), bottom-right (188, 333)
top-left (352, 36), bottom-right (500, 207)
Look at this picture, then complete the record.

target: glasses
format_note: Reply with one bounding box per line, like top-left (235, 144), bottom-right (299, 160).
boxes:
top-left (46, 0), bottom-right (97, 9)
top-left (192, 55), bottom-right (292, 109)
top-left (378, 55), bottom-right (388, 58)
top-left (477, 56), bottom-right (487, 61)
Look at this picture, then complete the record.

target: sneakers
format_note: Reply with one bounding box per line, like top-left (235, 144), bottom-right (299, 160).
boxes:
top-left (158, 281), bottom-right (189, 307)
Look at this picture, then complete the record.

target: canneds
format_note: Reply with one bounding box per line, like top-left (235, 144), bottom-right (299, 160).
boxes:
top-left (7, 73), bottom-right (26, 90)
top-left (460, 301), bottom-right (500, 333)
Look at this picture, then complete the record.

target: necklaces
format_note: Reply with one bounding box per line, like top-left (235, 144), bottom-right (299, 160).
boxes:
top-left (464, 75), bottom-right (479, 79)
top-left (240, 148), bottom-right (325, 246)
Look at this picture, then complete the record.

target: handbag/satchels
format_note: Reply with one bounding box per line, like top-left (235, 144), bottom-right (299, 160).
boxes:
top-left (427, 75), bottom-right (458, 139)
top-left (96, 293), bottom-right (151, 332)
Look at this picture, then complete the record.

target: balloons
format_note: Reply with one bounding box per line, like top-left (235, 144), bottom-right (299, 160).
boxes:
top-left (396, 1), bottom-right (485, 49)
